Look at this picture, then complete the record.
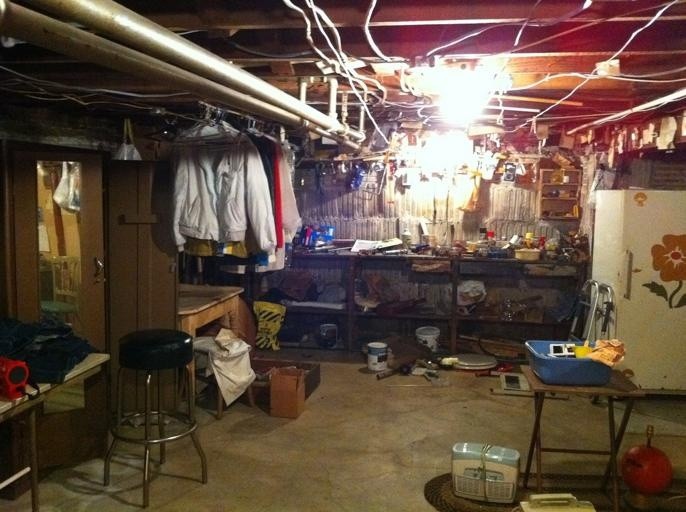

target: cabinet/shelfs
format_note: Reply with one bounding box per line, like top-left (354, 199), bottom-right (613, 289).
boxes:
top-left (591, 188), bottom-right (686, 397)
top-left (104, 154), bottom-right (182, 418)
top-left (247, 249), bottom-right (588, 356)
top-left (1, 355), bottom-right (110, 511)
top-left (537, 168), bottom-right (583, 222)
top-left (1, 140), bottom-right (109, 470)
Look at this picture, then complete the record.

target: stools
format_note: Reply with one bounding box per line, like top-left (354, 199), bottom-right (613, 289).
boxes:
top-left (104, 331), bottom-right (207, 507)
top-left (180, 337), bottom-right (258, 421)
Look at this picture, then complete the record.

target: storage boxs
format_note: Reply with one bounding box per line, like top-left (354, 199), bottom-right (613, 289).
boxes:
top-left (269, 368), bottom-right (305, 418)
top-left (451, 440), bottom-right (521, 504)
top-left (250, 358), bottom-right (321, 407)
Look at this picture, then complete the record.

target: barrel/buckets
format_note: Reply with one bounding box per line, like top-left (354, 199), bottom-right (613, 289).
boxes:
top-left (415, 325), bottom-right (441, 352)
top-left (367, 342), bottom-right (387, 371)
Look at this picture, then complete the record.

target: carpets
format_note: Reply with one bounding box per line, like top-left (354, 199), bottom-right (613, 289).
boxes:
top-left (424, 469), bottom-right (686, 512)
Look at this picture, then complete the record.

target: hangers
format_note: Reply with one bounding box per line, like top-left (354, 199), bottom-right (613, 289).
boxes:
top-left (173, 105), bottom-right (298, 154)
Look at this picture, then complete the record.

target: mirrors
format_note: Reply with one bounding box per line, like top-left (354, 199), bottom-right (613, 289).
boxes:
top-left (33, 159), bottom-right (85, 417)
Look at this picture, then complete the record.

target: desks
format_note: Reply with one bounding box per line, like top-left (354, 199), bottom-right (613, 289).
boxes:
top-left (519, 364), bottom-right (646, 512)
top-left (177, 284), bottom-right (245, 423)
